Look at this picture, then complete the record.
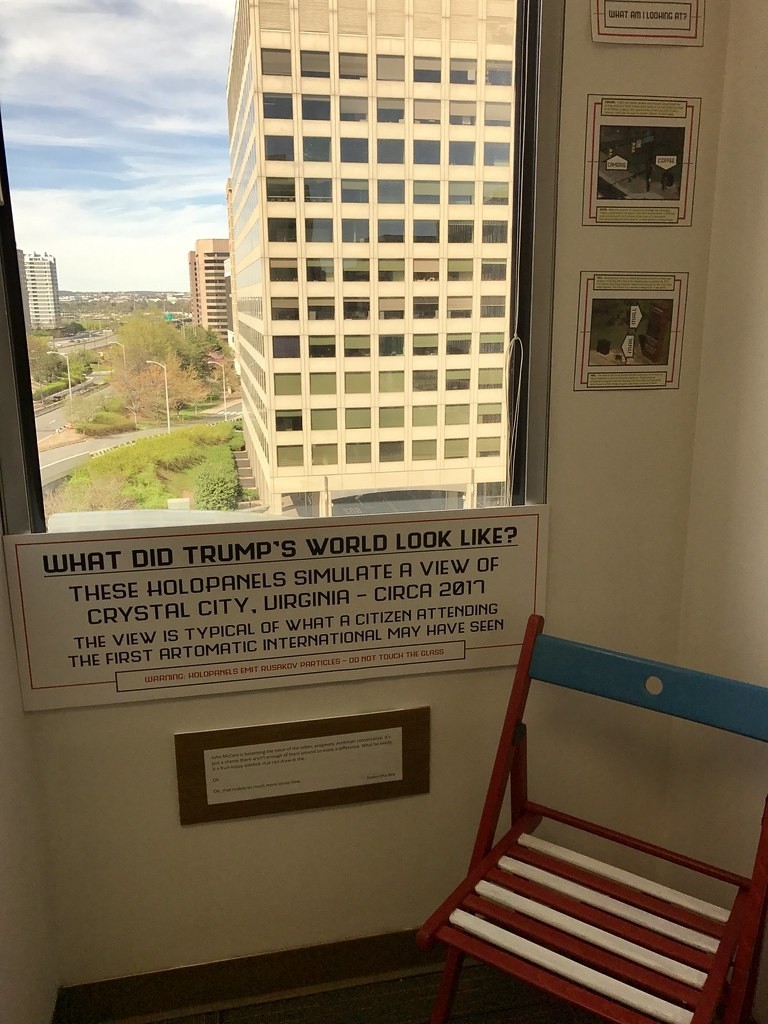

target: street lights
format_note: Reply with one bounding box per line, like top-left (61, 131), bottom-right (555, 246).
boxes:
top-left (108, 341), bottom-right (127, 384)
top-left (46, 351), bottom-right (73, 428)
top-left (207, 361), bottom-right (227, 421)
top-left (146, 360), bottom-right (170, 433)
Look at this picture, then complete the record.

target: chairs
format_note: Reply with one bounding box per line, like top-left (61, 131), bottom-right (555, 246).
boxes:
top-left (414, 613), bottom-right (768, 1024)
